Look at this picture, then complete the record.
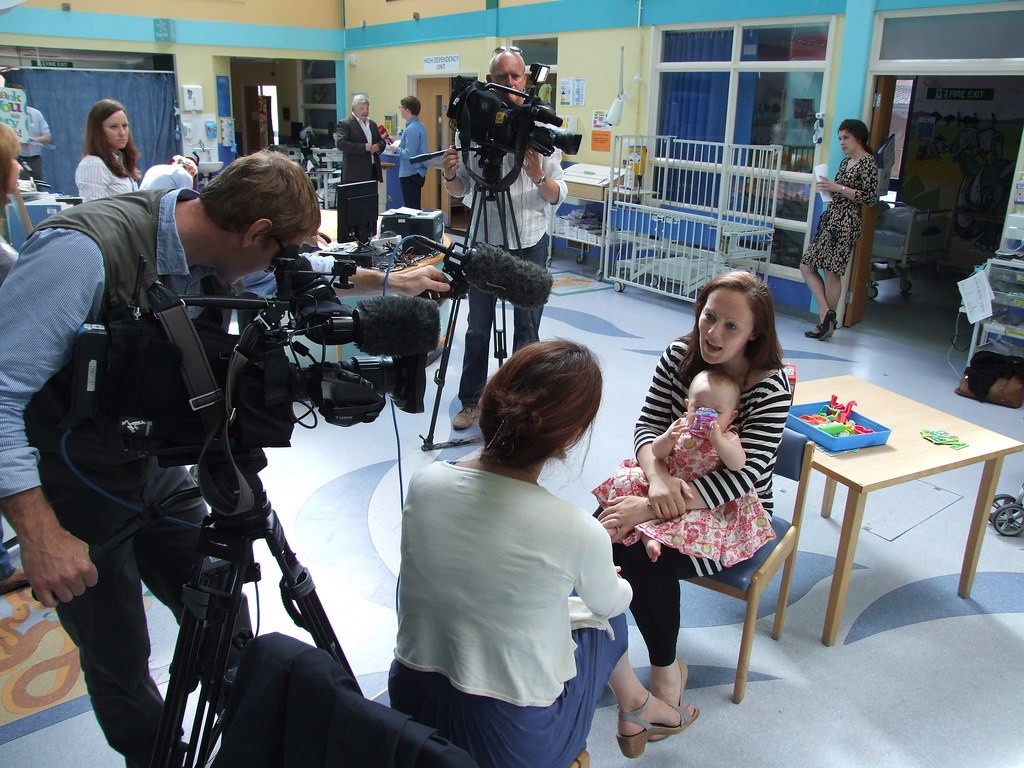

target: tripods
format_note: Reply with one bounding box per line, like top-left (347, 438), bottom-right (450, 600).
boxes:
top-left (409, 142), bottom-right (539, 450)
top-left (33, 438), bottom-right (363, 768)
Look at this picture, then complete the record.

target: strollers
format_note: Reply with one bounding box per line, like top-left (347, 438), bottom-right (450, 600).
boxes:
top-left (988, 482), bottom-right (1024, 535)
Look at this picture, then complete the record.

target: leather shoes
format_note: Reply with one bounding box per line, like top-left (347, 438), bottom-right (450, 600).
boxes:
top-left (616, 692), bottom-right (700, 758)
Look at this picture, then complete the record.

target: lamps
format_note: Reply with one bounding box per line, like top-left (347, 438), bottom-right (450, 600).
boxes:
top-left (605, 45), bottom-right (642, 127)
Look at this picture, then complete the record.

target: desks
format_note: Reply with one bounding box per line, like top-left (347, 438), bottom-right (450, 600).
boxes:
top-left (783, 374), bottom-right (1024, 647)
top-left (331, 232), bottom-right (452, 363)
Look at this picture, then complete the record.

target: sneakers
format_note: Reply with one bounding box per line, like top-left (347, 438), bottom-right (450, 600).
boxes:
top-left (453, 408), bottom-right (481, 429)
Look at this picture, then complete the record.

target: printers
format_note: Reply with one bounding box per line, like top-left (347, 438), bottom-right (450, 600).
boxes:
top-left (380, 208), bottom-right (444, 256)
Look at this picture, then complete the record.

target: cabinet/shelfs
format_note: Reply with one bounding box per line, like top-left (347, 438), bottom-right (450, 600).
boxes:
top-left (965, 257), bottom-right (1024, 368)
top-left (546, 195), bottom-right (639, 280)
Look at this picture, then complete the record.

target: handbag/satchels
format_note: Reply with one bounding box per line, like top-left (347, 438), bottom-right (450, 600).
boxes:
top-left (955, 373), bottom-right (1024, 407)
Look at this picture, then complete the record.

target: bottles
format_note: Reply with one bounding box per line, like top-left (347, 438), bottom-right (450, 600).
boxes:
top-left (624, 165), bottom-right (635, 189)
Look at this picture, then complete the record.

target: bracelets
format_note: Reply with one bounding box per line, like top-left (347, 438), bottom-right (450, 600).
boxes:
top-left (838, 186), bottom-right (846, 193)
top-left (647, 497), bottom-right (656, 517)
top-left (443, 172), bottom-right (456, 182)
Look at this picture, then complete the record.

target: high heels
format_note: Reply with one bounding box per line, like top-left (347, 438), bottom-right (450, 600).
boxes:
top-left (805, 324), bottom-right (836, 338)
top-left (819, 310), bottom-right (838, 340)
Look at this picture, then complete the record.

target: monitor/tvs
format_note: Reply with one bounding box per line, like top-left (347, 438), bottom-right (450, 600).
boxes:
top-left (291, 122), bottom-right (304, 145)
top-left (336, 180), bottom-right (380, 253)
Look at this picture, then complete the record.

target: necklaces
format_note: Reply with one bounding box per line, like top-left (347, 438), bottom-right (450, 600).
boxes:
top-left (506, 462), bottom-right (539, 479)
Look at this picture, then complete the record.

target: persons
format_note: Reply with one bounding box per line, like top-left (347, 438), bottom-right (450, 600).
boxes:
top-left (336, 94), bottom-right (428, 242)
top-left (593, 270), bottom-right (792, 711)
top-left (442, 46), bottom-right (569, 430)
top-left (800, 119), bottom-right (879, 341)
top-left (0, 151), bottom-right (320, 767)
top-left (387, 341), bottom-right (701, 768)
top-left (0, 83), bottom-right (200, 599)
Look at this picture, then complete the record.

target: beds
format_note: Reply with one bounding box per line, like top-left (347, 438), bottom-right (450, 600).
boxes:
top-left (866, 201), bottom-right (953, 298)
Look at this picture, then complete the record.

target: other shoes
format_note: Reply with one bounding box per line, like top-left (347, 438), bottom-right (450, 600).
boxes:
top-left (0, 569), bottom-right (29, 595)
top-left (648, 659), bottom-right (688, 741)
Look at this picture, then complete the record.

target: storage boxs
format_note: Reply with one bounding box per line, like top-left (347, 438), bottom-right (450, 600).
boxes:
top-left (556, 216), bottom-right (606, 246)
top-left (785, 401), bottom-right (891, 451)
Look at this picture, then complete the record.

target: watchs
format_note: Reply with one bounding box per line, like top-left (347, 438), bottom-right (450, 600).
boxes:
top-left (532, 175), bottom-right (547, 186)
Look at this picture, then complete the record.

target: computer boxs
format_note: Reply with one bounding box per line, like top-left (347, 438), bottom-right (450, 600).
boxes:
top-left (320, 244), bottom-right (392, 269)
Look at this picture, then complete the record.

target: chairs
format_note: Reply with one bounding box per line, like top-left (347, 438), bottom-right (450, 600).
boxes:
top-left (680, 425), bottom-right (815, 705)
top-left (208, 633), bottom-right (591, 768)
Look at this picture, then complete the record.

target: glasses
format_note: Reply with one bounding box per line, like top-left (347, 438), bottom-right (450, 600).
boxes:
top-left (399, 105), bottom-right (404, 109)
top-left (492, 46), bottom-right (523, 57)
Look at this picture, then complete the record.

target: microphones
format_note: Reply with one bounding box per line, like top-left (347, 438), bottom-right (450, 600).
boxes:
top-left (412, 235), bottom-right (555, 311)
top-left (377, 124), bottom-right (393, 145)
top-left (531, 108), bottom-right (564, 127)
top-left (322, 295), bottom-right (442, 359)
